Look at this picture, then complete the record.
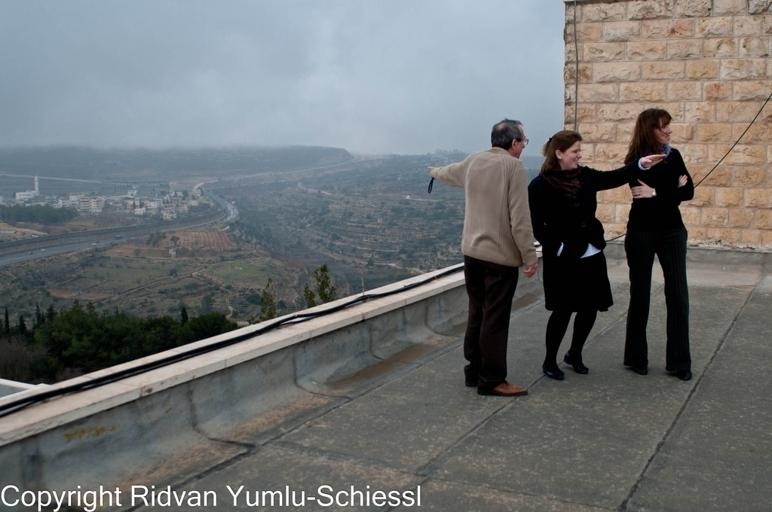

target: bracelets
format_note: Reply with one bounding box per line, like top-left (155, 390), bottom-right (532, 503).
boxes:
top-left (652, 187), bottom-right (656, 197)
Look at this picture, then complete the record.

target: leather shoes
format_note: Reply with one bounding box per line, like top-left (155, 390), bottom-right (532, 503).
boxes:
top-left (632, 365), bottom-right (647, 375)
top-left (478, 381), bottom-right (528, 396)
top-left (563, 349), bottom-right (589, 374)
top-left (542, 358), bottom-right (564, 380)
top-left (677, 368), bottom-right (691, 380)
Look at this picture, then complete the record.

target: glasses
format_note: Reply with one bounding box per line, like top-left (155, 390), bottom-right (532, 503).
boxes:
top-left (518, 140), bottom-right (529, 145)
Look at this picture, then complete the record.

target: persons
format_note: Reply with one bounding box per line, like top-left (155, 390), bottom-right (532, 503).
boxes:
top-left (426, 117), bottom-right (539, 397)
top-left (622, 107), bottom-right (697, 382)
top-left (528, 128), bottom-right (669, 381)
